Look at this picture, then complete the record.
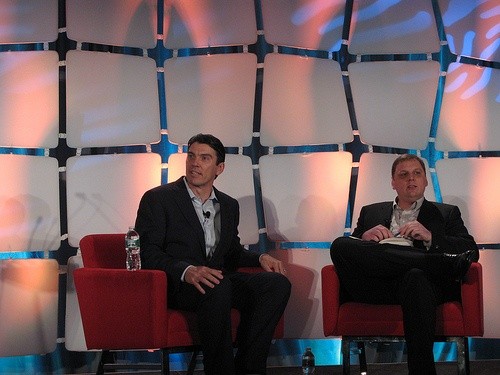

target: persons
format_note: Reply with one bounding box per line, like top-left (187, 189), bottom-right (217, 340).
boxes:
top-left (331, 154), bottom-right (480, 375)
top-left (134, 133), bottom-right (292, 375)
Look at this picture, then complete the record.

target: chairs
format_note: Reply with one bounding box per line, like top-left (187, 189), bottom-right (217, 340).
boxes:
top-left (73, 233), bottom-right (285, 375)
top-left (322, 262), bottom-right (483, 375)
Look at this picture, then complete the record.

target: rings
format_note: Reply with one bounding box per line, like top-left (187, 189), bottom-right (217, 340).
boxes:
top-left (283, 268), bottom-right (286, 272)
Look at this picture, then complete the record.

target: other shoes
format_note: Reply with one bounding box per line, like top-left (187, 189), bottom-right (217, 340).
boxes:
top-left (442, 250), bottom-right (477, 281)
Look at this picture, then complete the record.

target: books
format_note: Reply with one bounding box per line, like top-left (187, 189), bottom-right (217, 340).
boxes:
top-left (348, 236), bottom-right (414, 247)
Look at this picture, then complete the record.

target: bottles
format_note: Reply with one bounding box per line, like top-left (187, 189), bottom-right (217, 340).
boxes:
top-left (125, 225), bottom-right (141, 272)
top-left (301, 348), bottom-right (315, 375)
